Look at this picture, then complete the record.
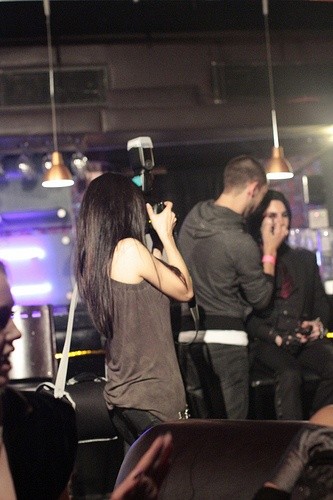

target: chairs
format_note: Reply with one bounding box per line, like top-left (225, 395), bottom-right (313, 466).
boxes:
top-left (113, 419), bottom-right (308, 500)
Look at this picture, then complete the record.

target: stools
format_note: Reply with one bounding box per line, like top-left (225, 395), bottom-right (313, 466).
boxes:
top-left (249, 371), bottom-right (321, 420)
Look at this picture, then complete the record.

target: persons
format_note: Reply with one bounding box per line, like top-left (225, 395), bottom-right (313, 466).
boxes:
top-left (0, 173), bottom-right (333, 500)
top-left (178, 156), bottom-right (290, 421)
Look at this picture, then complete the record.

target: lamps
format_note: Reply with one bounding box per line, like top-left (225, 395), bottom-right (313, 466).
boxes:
top-left (41, 0), bottom-right (74, 188)
top-left (16, 154), bottom-right (29, 169)
top-left (262, 0), bottom-right (295, 180)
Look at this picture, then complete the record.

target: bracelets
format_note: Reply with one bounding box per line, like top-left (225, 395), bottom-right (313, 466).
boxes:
top-left (262, 254), bottom-right (276, 264)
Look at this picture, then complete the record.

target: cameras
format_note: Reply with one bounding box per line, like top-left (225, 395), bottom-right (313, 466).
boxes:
top-left (126, 136), bottom-right (166, 234)
